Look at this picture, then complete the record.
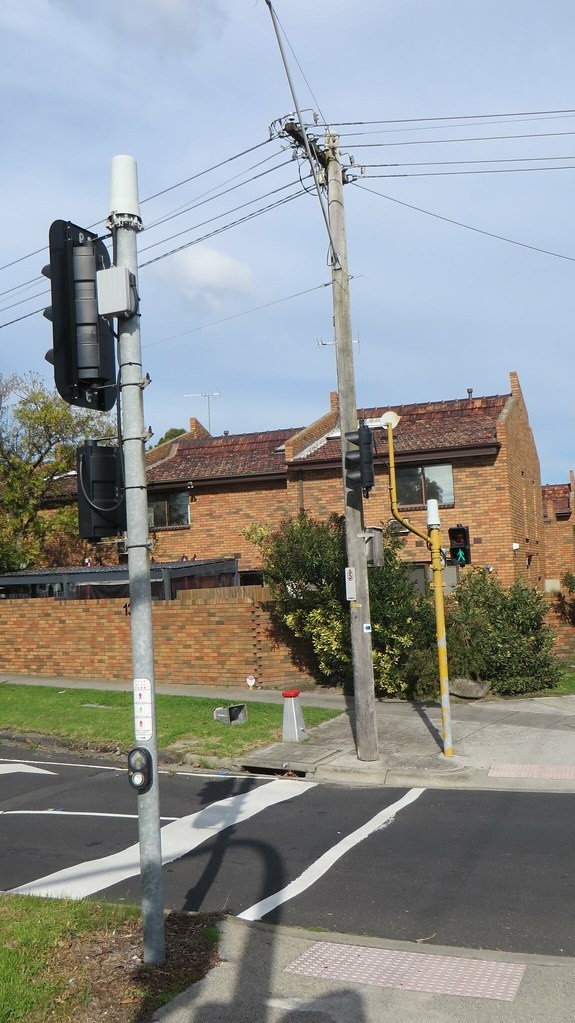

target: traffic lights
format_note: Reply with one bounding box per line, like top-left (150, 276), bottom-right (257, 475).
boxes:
top-left (449, 525), bottom-right (470, 566)
top-left (343, 425), bottom-right (378, 502)
top-left (39, 222), bottom-right (117, 410)
